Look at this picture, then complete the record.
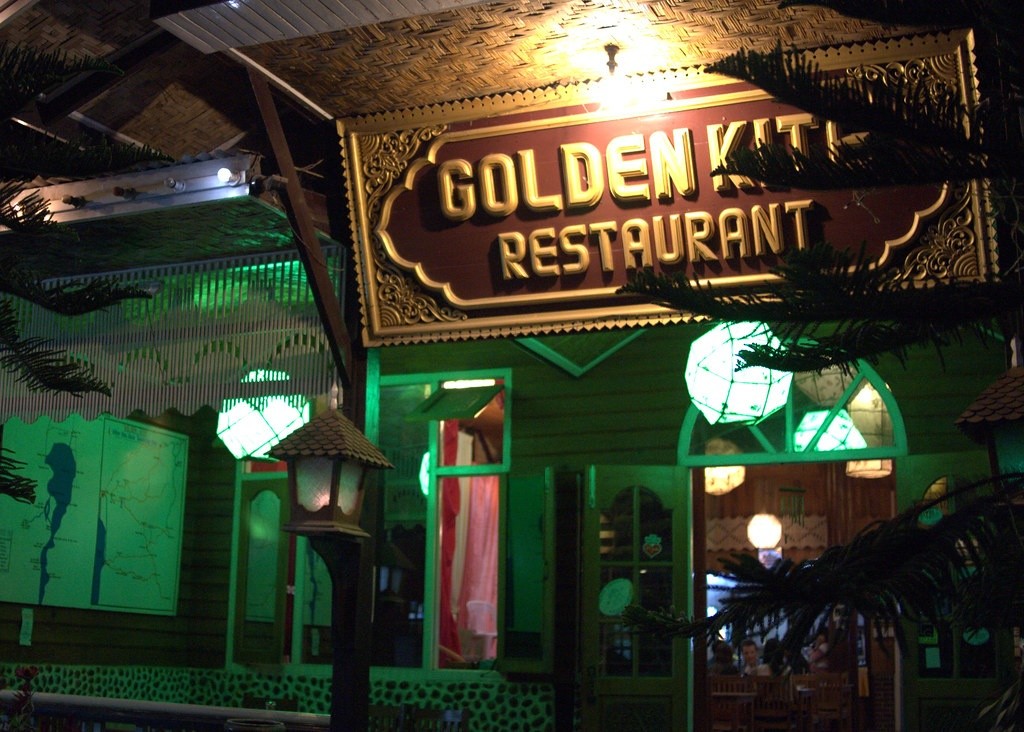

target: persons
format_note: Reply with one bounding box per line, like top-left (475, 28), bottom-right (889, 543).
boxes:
top-left (772, 631), bottom-right (844, 732)
top-left (739, 642), bottom-right (772, 692)
top-left (709, 641), bottom-right (737, 675)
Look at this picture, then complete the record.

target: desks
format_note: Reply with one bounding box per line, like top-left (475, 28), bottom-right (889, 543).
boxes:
top-left (0, 689), bottom-right (330, 732)
top-left (711, 692), bottom-right (758, 732)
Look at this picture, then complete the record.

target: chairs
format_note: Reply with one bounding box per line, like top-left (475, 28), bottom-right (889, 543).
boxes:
top-left (396, 703), bottom-right (471, 732)
top-left (367, 705), bottom-right (412, 732)
top-left (709, 672), bottom-right (852, 732)
top-left (241, 692), bottom-right (298, 712)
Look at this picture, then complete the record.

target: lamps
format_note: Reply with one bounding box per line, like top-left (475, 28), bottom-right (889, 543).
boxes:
top-left (792, 408), bottom-right (869, 453)
top-left (703, 437), bottom-right (746, 496)
top-left (746, 507), bottom-right (782, 550)
top-left (845, 382), bottom-right (892, 479)
top-left (952, 365), bottom-right (1024, 522)
top-left (683, 322), bottom-right (793, 427)
top-left (215, 369), bottom-right (310, 464)
top-left (375, 541), bottom-right (418, 610)
top-left (263, 407), bottom-right (395, 538)
